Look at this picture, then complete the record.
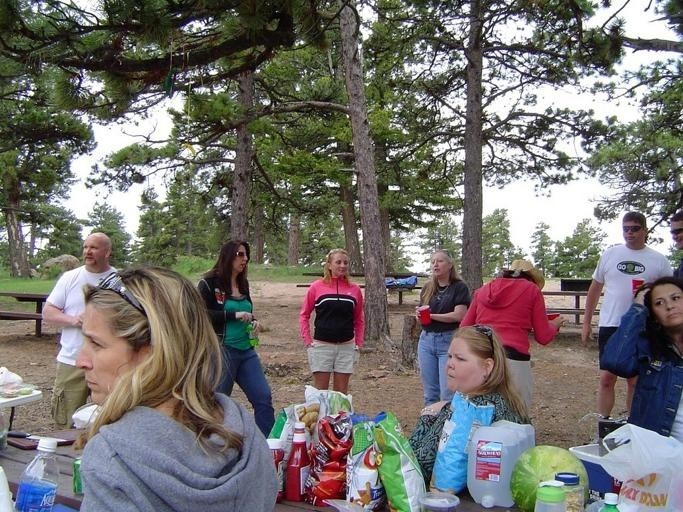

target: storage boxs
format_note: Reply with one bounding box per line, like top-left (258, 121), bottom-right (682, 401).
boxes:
top-left (569, 444), bottom-right (613, 493)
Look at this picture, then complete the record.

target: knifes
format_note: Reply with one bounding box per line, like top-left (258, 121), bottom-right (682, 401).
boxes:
top-left (7, 428), bottom-right (65, 441)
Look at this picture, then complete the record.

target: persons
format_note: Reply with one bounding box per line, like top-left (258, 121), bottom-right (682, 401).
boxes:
top-left (414, 251), bottom-right (470, 404)
top-left (42, 232), bottom-right (124, 428)
top-left (409, 323), bottom-right (534, 491)
top-left (299, 250), bottom-right (366, 394)
top-left (600, 277), bottom-right (683, 441)
top-left (460, 259), bottom-right (565, 420)
top-left (197, 241), bottom-right (274, 439)
top-left (74, 265), bottom-right (279, 512)
top-left (579, 213), bottom-right (675, 418)
top-left (670, 209), bottom-right (682, 277)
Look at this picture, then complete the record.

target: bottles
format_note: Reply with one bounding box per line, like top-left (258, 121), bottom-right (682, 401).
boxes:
top-left (285, 423), bottom-right (310, 503)
top-left (597, 491), bottom-right (621, 512)
top-left (265, 438), bottom-right (285, 501)
top-left (535, 480), bottom-right (567, 512)
top-left (551, 472), bottom-right (584, 512)
top-left (14, 437), bottom-right (61, 512)
top-left (246, 319), bottom-right (259, 346)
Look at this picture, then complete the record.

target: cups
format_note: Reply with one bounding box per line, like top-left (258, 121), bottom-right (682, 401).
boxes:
top-left (418, 305), bottom-right (431, 326)
top-left (417, 491), bottom-right (459, 512)
top-left (546, 313), bottom-right (559, 331)
top-left (631, 278), bottom-right (645, 295)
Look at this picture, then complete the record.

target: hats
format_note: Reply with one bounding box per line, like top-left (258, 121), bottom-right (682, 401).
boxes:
top-left (502, 260), bottom-right (545, 290)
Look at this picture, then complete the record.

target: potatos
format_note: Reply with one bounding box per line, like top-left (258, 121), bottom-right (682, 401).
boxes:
top-left (297, 402), bottom-right (320, 435)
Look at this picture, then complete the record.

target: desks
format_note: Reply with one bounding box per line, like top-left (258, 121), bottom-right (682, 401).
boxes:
top-left (0, 292), bottom-right (48, 337)
top-left (542, 292), bottom-right (604, 324)
top-left (0, 429), bottom-right (522, 512)
top-left (303, 272), bottom-right (429, 306)
top-left (0, 388), bottom-right (43, 431)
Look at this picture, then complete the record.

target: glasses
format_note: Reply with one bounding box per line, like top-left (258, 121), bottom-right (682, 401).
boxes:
top-left (624, 225), bottom-right (641, 233)
top-left (98, 272), bottom-right (147, 320)
top-left (670, 227), bottom-right (683, 234)
top-left (235, 252), bottom-right (244, 257)
top-left (474, 323), bottom-right (494, 356)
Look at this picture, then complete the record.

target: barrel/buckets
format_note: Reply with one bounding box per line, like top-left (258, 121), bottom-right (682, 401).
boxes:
top-left (466, 420), bottom-right (536, 508)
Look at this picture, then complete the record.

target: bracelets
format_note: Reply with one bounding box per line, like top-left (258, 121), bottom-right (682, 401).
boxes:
top-left (421, 405), bottom-right (439, 417)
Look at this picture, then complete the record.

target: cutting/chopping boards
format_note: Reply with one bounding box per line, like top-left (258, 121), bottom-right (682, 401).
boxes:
top-left (6, 436), bottom-right (75, 449)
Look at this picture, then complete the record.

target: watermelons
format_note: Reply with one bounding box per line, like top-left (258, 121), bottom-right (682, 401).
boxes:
top-left (510, 445), bottom-right (590, 512)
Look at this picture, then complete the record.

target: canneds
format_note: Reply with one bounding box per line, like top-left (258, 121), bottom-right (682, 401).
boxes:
top-left (73, 456), bottom-right (83, 495)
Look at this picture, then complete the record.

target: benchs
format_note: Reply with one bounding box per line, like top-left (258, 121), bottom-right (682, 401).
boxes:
top-left (546, 308), bottom-right (599, 324)
top-left (297, 284), bottom-right (422, 306)
top-left (0, 312), bottom-right (44, 338)
